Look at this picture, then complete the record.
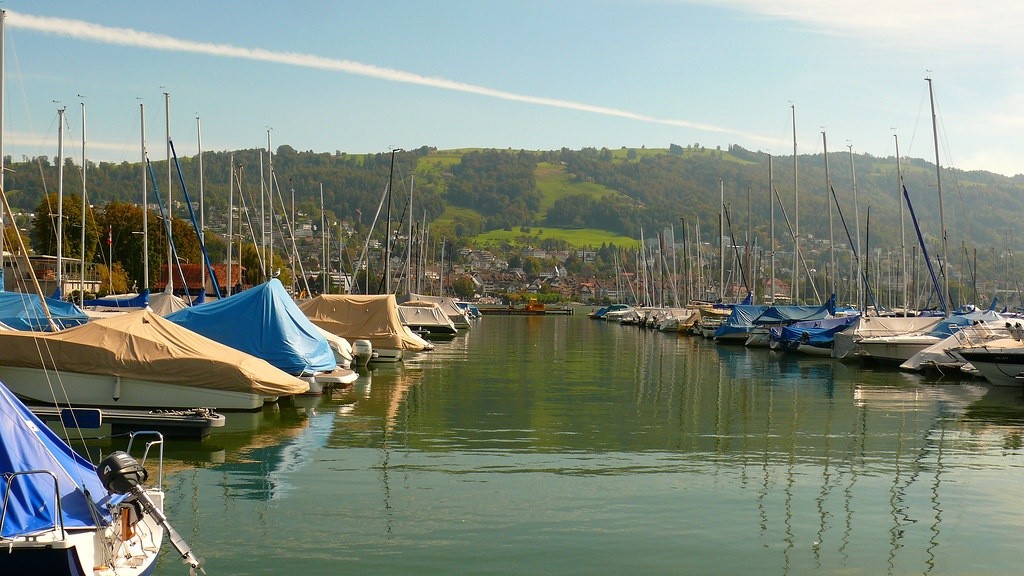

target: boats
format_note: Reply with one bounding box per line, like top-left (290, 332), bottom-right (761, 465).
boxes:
top-left (506, 297), bottom-right (546, 316)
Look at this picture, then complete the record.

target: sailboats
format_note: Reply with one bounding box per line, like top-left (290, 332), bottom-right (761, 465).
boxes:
top-left (1, 8), bottom-right (484, 576)
top-left (586, 67), bottom-right (1024, 385)
